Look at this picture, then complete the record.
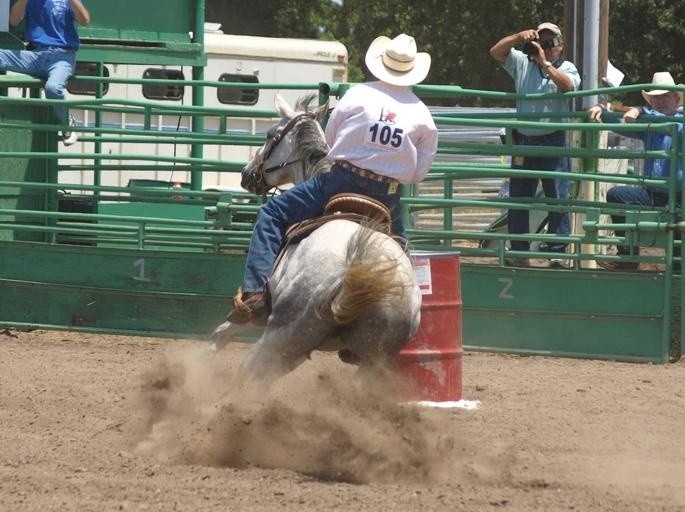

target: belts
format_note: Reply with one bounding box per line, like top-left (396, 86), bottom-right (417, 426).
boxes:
top-left (337, 160), bottom-right (392, 184)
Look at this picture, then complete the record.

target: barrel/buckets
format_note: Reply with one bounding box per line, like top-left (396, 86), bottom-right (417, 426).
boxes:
top-left (379, 249), bottom-right (465, 403)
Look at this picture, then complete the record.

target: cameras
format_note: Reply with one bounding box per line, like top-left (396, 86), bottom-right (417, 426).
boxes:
top-left (522, 37), bottom-right (560, 54)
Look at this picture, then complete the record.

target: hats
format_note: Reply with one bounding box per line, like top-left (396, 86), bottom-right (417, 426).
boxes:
top-left (537, 22), bottom-right (562, 38)
top-left (364, 33), bottom-right (431, 87)
top-left (642, 72), bottom-right (684, 108)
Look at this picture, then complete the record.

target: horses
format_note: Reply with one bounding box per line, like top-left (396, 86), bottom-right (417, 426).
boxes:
top-left (206, 92), bottom-right (423, 465)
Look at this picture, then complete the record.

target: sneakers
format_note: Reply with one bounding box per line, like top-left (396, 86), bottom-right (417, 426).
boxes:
top-left (62, 113), bottom-right (78, 145)
top-left (549, 259), bottom-right (565, 267)
top-left (227, 292), bottom-right (272, 324)
top-left (505, 257), bottom-right (529, 266)
top-left (596, 260), bottom-right (639, 270)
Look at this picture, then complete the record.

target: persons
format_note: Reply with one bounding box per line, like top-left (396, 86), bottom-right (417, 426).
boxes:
top-left (586, 72), bottom-right (685, 273)
top-left (227, 32), bottom-right (439, 325)
top-left (0, 0), bottom-right (90, 146)
top-left (490, 22), bottom-right (582, 267)
